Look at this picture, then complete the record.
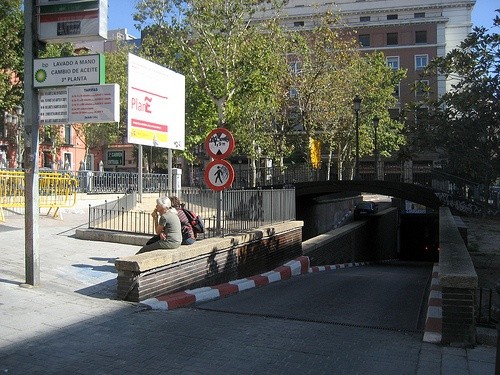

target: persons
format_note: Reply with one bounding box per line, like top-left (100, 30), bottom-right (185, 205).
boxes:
top-left (179, 203), bottom-right (198, 239)
top-left (134, 196), bottom-right (183, 256)
top-left (144, 196), bottom-right (196, 246)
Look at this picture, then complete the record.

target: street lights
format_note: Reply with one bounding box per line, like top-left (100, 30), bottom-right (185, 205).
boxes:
top-left (351, 94), bottom-right (362, 180)
top-left (371, 114), bottom-right (380, 181)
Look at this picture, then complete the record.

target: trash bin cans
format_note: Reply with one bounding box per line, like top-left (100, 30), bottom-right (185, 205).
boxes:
top-left (79, 172), bottom-right (93, 192)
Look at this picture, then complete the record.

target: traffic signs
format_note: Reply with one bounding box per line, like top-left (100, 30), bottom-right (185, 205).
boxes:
top-left (32, 52), bottom-right (121, 126)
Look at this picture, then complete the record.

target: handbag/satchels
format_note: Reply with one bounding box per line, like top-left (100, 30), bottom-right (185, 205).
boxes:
top-left (181, 208), bottom-right (205, 233)
